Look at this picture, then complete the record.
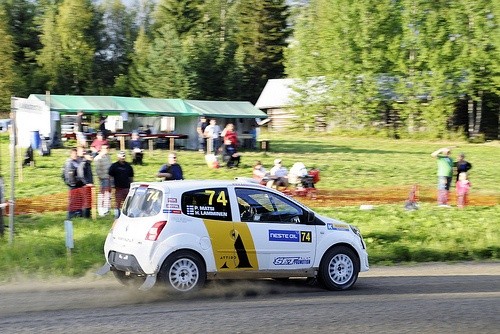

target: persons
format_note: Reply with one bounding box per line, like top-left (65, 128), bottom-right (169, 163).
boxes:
top-left (153, 150), bottom-right (185, 180)
top-left (430, 147), bottom-right (473, 209)
top-left (251, 158), bottom-right (291, 192)
top-left (137, 124), bottom-right (153, 146)
top-left (127, 129), bottom-right (145, 165)
top-left (196, 116), bottom-right (242, 169)
top-left (89, 131), bottom-right (110, 158)
top-left (61, 143), bottom-right (135, 221)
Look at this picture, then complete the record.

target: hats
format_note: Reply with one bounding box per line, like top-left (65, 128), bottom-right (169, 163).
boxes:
top-left (97, 132), bottom-right (103, 136)
top-left (117, 150), bottom-right (126, 158)
top-left (274, 159), bottom-right (281, 163)
top-left (83, 149), bottom-right (92, 155)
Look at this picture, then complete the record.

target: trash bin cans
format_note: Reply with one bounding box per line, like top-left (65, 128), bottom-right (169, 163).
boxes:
top-left (259, 140), bottom-right (269, 152)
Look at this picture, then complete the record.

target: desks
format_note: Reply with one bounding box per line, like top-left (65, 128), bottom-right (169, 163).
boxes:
top-left (257, 138), bottom-right (271, 151)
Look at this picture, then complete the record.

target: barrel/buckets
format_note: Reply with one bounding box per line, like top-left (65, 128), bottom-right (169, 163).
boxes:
top-left (29, 131), bottom-right (40, 150)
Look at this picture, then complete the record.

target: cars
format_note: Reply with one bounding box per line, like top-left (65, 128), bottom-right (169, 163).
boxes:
top-left (104, 176), bottom-right (370, 299)
